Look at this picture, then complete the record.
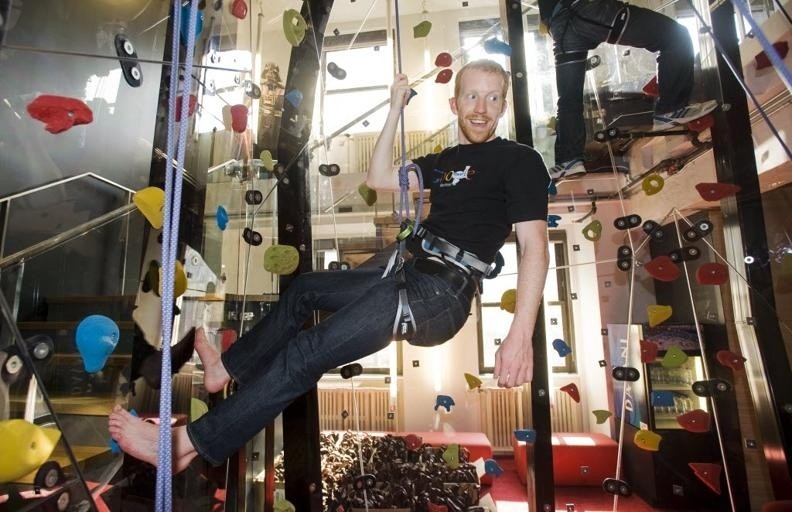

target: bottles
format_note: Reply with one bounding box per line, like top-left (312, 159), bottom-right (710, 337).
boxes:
top-left (648, 365), bottom-right (693, 414)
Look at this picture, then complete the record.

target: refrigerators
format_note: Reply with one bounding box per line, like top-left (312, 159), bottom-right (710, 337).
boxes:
top-left (605, 322), bottom-right (751, 512)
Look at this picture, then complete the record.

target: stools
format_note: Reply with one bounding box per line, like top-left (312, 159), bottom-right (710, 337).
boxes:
top-left (511, 430), bottom-right (619, 489)
top-left (396, 432), bottom-right (494, 486)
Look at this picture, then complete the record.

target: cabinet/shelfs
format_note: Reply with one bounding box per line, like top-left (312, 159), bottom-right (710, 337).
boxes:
top-left (607, 320), bottom-right (749, 512)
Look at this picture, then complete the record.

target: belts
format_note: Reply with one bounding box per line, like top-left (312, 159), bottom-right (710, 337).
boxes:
top-left (414, 259), bottom-right (474, 301)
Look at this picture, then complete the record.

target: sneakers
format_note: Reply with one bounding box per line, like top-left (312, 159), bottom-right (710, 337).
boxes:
top-left (653, 99), bottom-right (719, 131)
top-left (548, 158), bottom-right (587, 178)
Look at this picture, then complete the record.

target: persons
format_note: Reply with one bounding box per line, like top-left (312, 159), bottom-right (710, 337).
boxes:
top-left (0, 101), bottom-right (197, 395)
top-left (536, 1), bottom-right (722, 182)
top-left (107, 57), bottom-right (552, 475)
